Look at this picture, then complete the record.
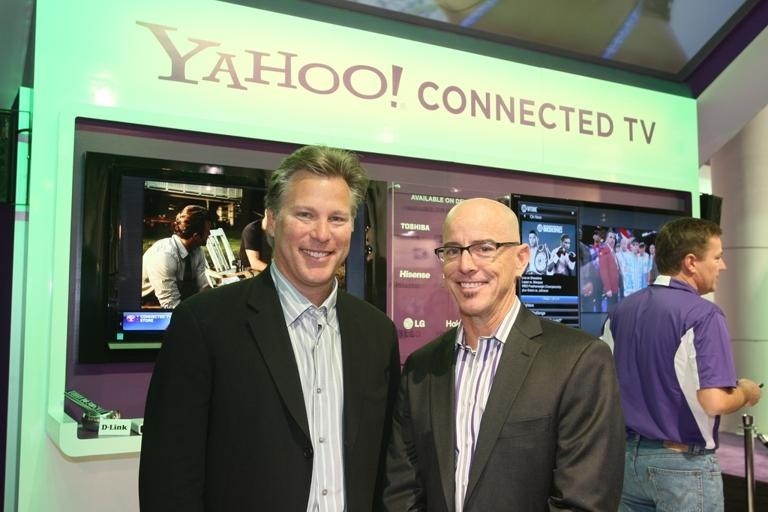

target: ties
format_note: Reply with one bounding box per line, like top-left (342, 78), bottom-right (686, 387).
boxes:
top-left (183, 254), bottom-right (193, 297)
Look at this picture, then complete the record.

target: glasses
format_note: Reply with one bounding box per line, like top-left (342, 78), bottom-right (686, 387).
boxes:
top-left (433, 241), bottom-right (521, 265)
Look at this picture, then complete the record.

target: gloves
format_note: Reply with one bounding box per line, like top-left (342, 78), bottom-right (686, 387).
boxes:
top-left (557, 247), bottom-right (566, 257)
top-left (569, 251), bottom-right (577, 262)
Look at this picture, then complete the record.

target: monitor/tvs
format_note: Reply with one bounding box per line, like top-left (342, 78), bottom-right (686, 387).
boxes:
top-left (508, 191), bottom-right (692, 337)
top-left (103, 165), bottom-right (365, 355)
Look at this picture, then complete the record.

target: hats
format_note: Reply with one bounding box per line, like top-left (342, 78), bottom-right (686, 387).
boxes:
top-left (630, 236), bottom-right (645, 246)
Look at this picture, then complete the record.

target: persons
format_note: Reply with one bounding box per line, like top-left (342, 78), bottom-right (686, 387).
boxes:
top-left (141, 203), bottom-right (213, 314)
top-left (235, 199), bottom-right (273, 278)
top-left (548, 233), bottom-right (575, 275)
top-left (605, 215), bottom-right (761, 512)
top-left (383, 196), bottom-right (628, 511)
top-left (527, 230), bottom-right (545, 275)
top-left (137, 143), bottom-right (408, 512)
top-left (579, 223), bottom-right (659, 310)
